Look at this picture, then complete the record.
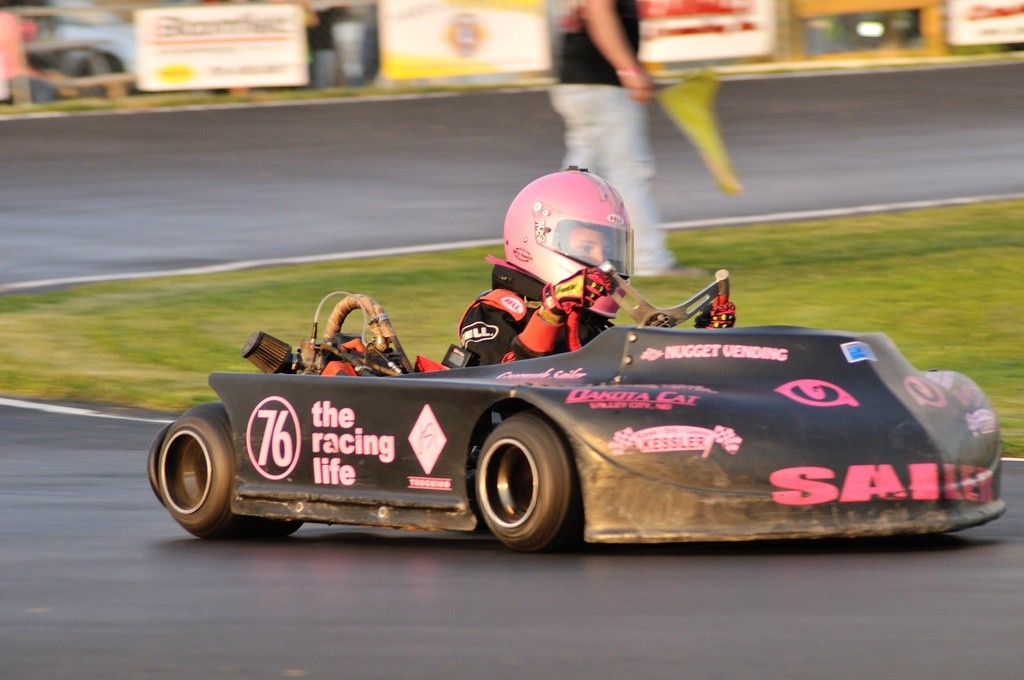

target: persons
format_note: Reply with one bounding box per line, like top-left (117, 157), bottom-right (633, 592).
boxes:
top-left (546, 0), bottom-right (710, 278)
top-left (459, 167), bottom-right (737, 367)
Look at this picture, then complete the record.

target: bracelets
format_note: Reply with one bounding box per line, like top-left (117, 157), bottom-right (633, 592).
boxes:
top-left (615, 66), bottom-right (644, 77)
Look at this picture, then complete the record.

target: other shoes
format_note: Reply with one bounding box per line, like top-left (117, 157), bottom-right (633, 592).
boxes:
top-left (633, 254), bottom-right (708, 277)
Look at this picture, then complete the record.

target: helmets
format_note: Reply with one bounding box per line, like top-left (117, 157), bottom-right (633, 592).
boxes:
top-left (504, 166), bottom-right (633, 317)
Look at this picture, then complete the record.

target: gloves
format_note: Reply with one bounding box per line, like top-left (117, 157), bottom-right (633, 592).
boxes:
top-left (693, 296), bottom-right (736, 330)
top-left (542, 268), bottom-right (625, 317)
